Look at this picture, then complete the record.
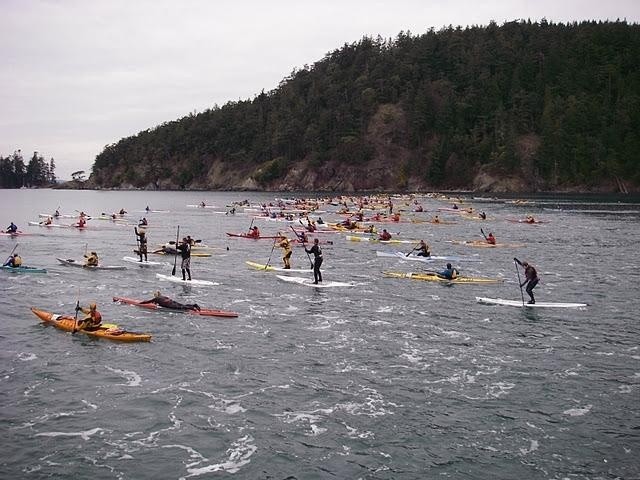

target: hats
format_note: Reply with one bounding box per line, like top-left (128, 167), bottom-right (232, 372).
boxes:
top-left (90, 304), bottom-right (96, 309)
top-left (154, 290), bottom-right (161, 298)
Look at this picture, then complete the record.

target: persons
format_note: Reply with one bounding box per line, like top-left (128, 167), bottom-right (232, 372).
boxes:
top-left (41, 208), bottom-right (126, 228)
top-left (437, 264), bottom-right (460, 279)
top-left (226, 194), bottom-right (399, 240)
top-left (200, 202), bottom-right (205, 207)
top-left (487, 232), bottom-right (498, 244)
top-left (134, 206), bottom-right (153, 262)
top-left (75, 304), bottom-right (104, 330)
top-left (415, 240), bottom-right (431, 256)
top-left (137, 291), bottom-right (202, 310)
top-left (275, 233), bottom-right (293, 269)
top-left (399, 192), bottom-right (439, 223)
top-left (84, 252), bottom-right (99, 266)
top-left (4, 253), bottom-right (23, 269)
top-left (514, 258), bottom-right (539, 303)
top-left (176, 235), bottom-right (196, 281)
top-left (304, 239), bottom-right (325, 284)
top-left (6, 222), bottom-right (17, 234)
top-left (445, 195), bottom-right (528, 220)
top-left (525, 214), bottom-right (536, 222)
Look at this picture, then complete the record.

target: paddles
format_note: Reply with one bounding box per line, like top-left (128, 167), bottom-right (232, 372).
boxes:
top-left (290, 226), bottom-right (313, 270)
top-left (406, 244), bottom-right (419, 257)
top-left (171, 226), bottom-right (179, 275)
top-left (265, 232), bottom-right (277, 270)
top-left (481, 228), bottom-right (488, 240)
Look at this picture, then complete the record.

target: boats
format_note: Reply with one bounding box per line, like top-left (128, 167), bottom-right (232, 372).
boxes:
top-left (186, 194), bottom-right (547, 264)
top-left (0, 264), bottom-right (47, 274)
top-left (121, 256), bottom-right (167, 268)
top-left (55, 257), bottom-right (127, 271)
top-left (28, 222), bottom-right (69, 228)
top-left (113, 296), bottom-right (240, 319)
top-left (30, 307), bottom-right (152, 344)
top-left (0, 230), bottom-right (33, 237)
